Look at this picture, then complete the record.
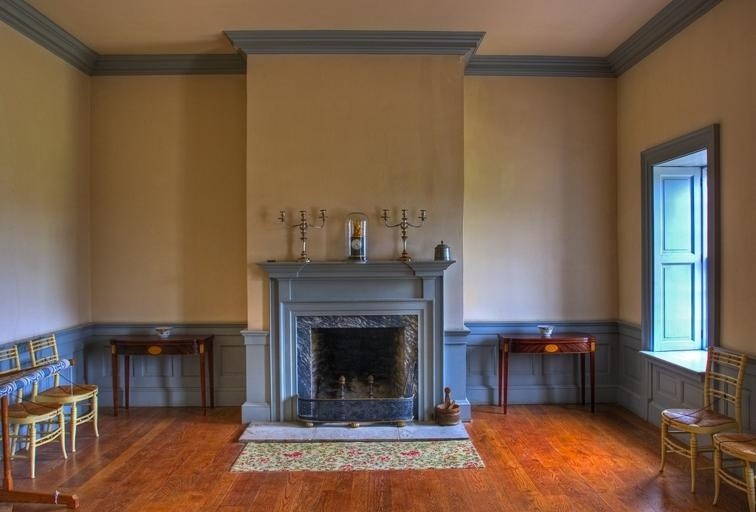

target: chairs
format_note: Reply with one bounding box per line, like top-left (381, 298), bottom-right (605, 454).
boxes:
top-left (660, 347), bottom-right (755, 512)
top-left (0, 334), bottom-right (99, 477)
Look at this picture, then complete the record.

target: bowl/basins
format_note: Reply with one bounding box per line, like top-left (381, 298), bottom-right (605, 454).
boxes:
top-left (537, 326), bottom-right (554, 337)
top-left (156, 327), bottom-right (172, 338)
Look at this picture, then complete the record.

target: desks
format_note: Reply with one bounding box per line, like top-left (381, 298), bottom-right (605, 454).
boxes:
top-left (497, 334), bottom-right (595, 414)
top-left (109, 334), bottom-right (214, 417)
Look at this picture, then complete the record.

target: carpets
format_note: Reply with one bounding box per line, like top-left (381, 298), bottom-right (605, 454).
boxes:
top-left (227, 438), bottom-right (487, 471)
